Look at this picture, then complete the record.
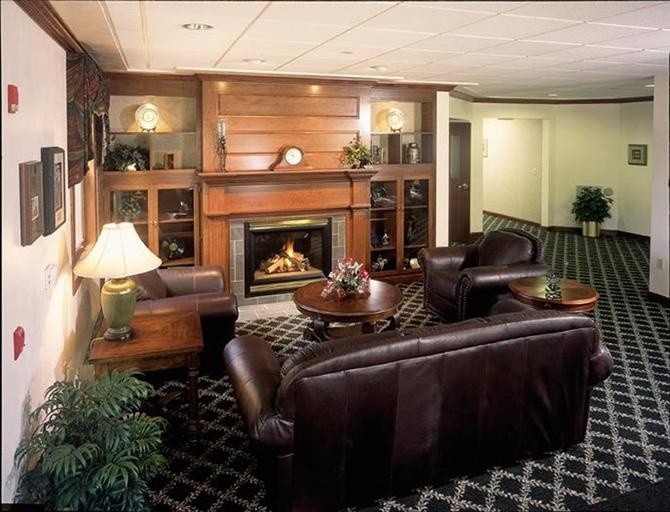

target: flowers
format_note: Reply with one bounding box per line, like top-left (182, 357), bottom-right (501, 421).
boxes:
top-left (320, 257), bottom-right (372, 301)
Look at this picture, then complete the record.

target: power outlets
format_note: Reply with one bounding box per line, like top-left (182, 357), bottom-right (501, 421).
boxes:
top-left (628, 144), bottom-right (648, 165)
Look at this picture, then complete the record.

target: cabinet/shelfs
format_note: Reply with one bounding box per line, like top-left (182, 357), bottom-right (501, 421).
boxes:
top-left (370, 80), bottom-right (436, 285)
top-left (191, 72), bottom-right (377, 180)
top-left (80, 73), bottom-right (198, 268)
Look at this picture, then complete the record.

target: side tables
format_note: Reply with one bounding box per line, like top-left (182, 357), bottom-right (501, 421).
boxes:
top-left (506, 275), bottom-right (600, 313)
top-left (89, 309), bottom-right (204, 420)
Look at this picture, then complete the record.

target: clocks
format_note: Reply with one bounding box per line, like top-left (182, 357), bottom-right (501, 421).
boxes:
top-left (269, 143), bottom-right (304, 171)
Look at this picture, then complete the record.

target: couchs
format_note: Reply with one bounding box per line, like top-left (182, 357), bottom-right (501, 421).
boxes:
top-left (417, 228), bottom-right (548, 323)
top-left (87, 265), bottom-right (237, 345)
top-left (221, 299), bottom-right (614, 511)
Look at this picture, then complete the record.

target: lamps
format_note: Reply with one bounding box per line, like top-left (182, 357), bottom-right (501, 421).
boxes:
top-left (72, 221), bottom-right (162, 340)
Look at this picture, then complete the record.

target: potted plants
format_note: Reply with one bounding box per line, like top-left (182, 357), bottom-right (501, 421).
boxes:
top-left (569, 186), bottom-right (615, 237)
top-left (339, 135), bottom-right (375, 169)
top-left (103, 136), bottom-right (149, 172)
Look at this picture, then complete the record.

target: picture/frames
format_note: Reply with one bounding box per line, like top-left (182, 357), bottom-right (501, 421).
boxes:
top-left (41, 145), bottom-right (66, 236)
top-left (19, 160), bottom-right (45, 246)
